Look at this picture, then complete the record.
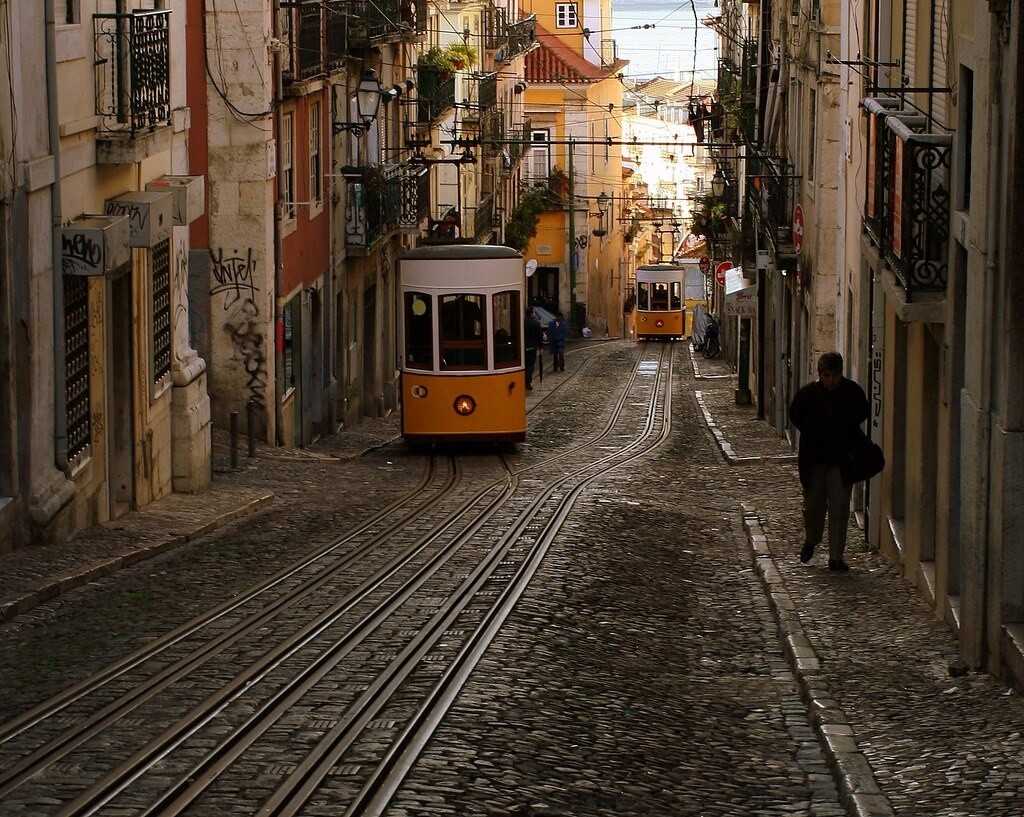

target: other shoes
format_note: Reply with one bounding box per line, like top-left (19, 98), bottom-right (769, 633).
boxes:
top-left (553, 364), bottom-right (558, 371)
top-left (801, 537), bottom-right (823, 564)
top-left (828, 558), bottom-right (851, 571)
top-left (525, 383), bottom-right (533, 390)
top-left (560, 365), bottom-right (564, 371)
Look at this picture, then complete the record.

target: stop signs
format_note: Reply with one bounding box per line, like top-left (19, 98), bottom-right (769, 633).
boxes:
top-left (793, 204), bottom-right (804, 255)
top-left (715, 261), bottom-right (734, 288)
top-left (699, 257), bottom-right (710, 273)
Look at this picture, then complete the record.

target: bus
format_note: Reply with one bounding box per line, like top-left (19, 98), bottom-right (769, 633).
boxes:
top-left (394, 139), bottom-right (529, 456)
top-left (635, 222), bottom-right (687, 343)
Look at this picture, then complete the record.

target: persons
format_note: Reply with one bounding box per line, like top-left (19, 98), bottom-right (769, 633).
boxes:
top-left (654, 285), bottom-right (669, 310)
top-left (790, 352), bottom-right (869, 568)
top-left (495, 329), bottom-right (516, 362)
top-left (548, 312), bottom-right (569, 371)
top-left (524, 307), bottom-right (544, 391)
top-left (407, 295), bottom-right (482, 367)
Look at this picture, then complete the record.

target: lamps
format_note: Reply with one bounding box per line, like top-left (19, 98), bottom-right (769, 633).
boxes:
top-left (332, 66), bottom-right (381, 138)
top-left (711, 169), bottom-right (726, 205)
top-left (589, 192), bottom-right (610, 218)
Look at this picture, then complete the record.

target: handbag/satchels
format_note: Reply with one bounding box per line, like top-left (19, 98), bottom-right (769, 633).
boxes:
top-left (837, 433), bottom-right (885, 486)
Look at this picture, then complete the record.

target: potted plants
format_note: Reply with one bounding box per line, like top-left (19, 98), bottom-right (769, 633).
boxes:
top-left (417, 42), bottom-right (478, 78)
top-left (444, 50), bottom-right (471, 70)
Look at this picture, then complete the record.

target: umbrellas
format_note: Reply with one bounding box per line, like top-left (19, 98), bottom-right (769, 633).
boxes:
top-left (538, 347), bottom-right (544, 387)
